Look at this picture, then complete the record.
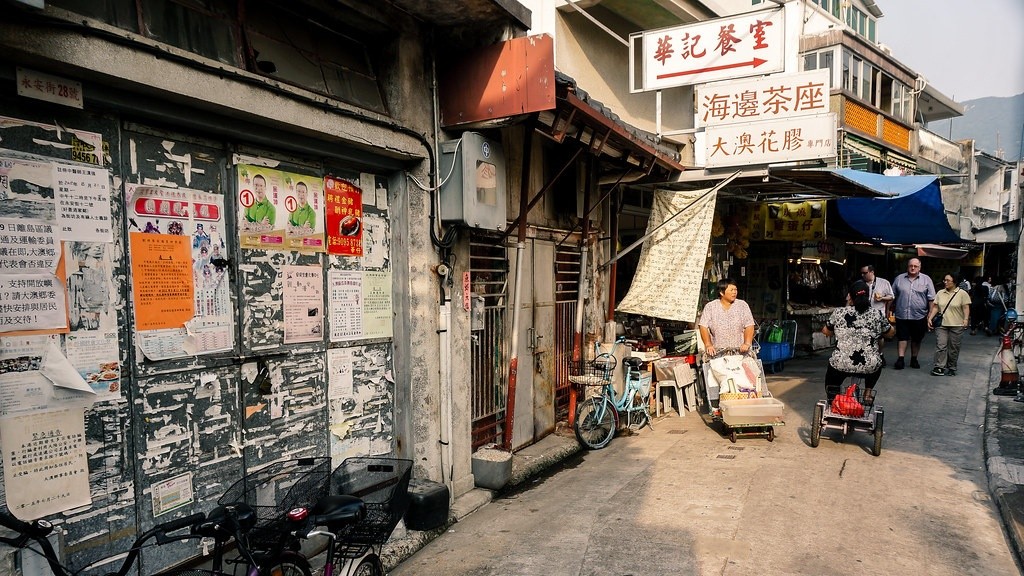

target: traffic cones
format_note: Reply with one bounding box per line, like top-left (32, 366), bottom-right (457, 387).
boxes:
top-left (993, 334), bottom-right (1024, 396)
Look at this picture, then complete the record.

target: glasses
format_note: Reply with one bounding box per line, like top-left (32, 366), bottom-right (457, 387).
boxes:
top-left (860, 272), bottom-right (868, 275)
top-left (943, 279), bottom-right (953, 282)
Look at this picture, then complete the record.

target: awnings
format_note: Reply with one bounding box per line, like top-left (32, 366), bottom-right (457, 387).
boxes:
top-left (826, 167), bottom-right (962, 243)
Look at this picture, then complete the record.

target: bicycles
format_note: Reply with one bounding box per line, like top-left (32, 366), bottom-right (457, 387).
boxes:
top-left (1, 455), bottom-right (416, 576)
top-left (567, 336), bottom-right (655, 451)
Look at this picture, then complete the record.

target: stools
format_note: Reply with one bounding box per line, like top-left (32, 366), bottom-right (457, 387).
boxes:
top-left (655, 368), bottom-right (703, 418)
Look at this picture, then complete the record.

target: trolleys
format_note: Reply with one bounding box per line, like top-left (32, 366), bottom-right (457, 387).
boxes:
top-left (706, 345), bottom-right (785, 443)
top-left (752, 318), bottom-right (798, 373)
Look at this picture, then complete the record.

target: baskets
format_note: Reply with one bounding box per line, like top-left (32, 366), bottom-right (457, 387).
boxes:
top-left (720, 392), bottom-right (757, 402)
top-left (826, 384), bottom-right (877, 417)
top-left (217, 456), bottom-right (415, 546)
top-left (568, 359), bottom-right (614, 384)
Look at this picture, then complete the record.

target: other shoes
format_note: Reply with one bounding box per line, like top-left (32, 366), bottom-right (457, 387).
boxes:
top-left (983, 327), bottom-right (988, 332)
top-left (970, 331), bottom-right (976, 336)
top-left (964, 328), bottom-right (967, 330)
top-left (986, 331), bottom-right (991, 337)
top-left (894, 359), bottom-right (905, 369)
top-left (863, 394), bottom-right (875, 403)
top-left (910, 360), bottom-right (920, 369)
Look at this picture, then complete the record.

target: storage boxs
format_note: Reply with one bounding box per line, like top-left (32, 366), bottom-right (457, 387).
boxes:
top-left (755, 342), bottom-right (790, 360)
top-left (631, 348), bottom-right (687, 381)
top-left (719, 398), bottom-right (785, 425)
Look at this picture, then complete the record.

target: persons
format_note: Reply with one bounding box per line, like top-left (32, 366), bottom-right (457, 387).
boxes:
top-left (860, 263), bottom-right (895, 318)
top-left (928, 274), bottom-right (972, 377)
top-left (286, 181), bottom-right (316, 235)
top-left (243, 174), bottom-right (276, 229)
top-left (887, 258), bottom-right (935, 370)
top-left (822, 280), bottom-right (896, 406)
top-left (698, 278), bottom-right (756, 359)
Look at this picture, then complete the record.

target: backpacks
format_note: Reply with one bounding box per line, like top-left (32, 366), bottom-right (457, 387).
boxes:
top-left (959, 281), bottom-right (968, 291)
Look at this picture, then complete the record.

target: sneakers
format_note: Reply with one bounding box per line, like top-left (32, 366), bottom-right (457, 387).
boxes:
top-left (947, 369), bottom-right (956, 376)
top-left (932, 367), bottom-right (944, 375)
top-left (880, 354), bottom-right (887, 366)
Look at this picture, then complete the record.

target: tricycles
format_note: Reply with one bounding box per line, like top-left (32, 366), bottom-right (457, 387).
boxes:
top-left (810, 377), bottom-right (887, 457)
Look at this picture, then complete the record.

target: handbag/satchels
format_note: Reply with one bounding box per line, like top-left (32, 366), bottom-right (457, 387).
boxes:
top-left (989, 284), bottom-right (1008, 306)
top-left (930, 312), bottom-right (943, 330)
top-left (832, 384), bottom-right (865, 416)
